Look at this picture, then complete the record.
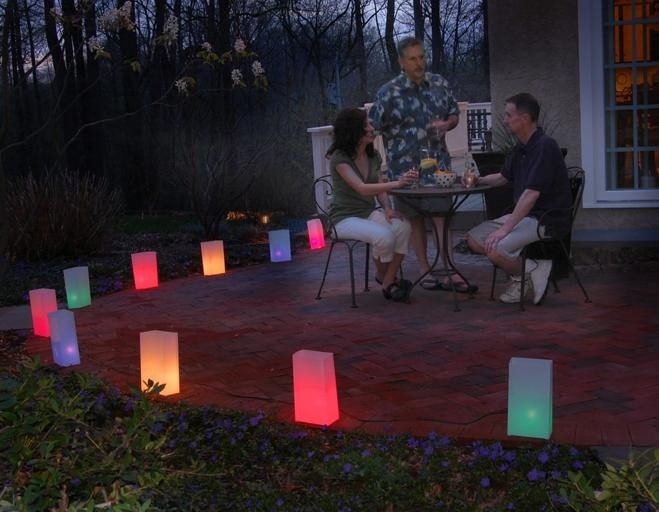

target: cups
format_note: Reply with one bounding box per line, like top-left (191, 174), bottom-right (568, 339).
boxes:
top-left (463, 161), bottom-right (476, 189)
top-left (411, 166), bottom-right (420, 188)
top-left (430, 114), bottom-right (442, 137)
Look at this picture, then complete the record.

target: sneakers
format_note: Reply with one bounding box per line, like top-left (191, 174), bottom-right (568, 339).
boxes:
top-left (529, 256), bottom-right (554, 306)
top-left (416, 270), bottom-right (438, 290)
top-left (440, 272), bottom-right (479, 292)
top-left (499, 273), bottom-right (529, 304)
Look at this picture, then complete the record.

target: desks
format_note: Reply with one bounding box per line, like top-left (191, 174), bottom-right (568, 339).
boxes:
top-left (386, 178), bottom-right (501, 311)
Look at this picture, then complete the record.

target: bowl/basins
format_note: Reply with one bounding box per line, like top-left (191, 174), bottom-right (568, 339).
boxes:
top-left (433, 173), bottom-right (457, 189)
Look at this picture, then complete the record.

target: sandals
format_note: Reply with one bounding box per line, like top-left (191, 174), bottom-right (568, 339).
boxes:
top-left (375, 275), bottom-right (414, 290)
top-left (382, 282), bottom-right (407, 302)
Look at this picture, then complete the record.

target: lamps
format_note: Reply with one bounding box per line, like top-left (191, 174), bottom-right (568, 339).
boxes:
top-left (139, 330), bottom-right (179, 396)
top-left (508, 357), bottom-right (556, 438)
top-left (131, 251), bottom-right (158, 289)
top-left (30, 265), bottom-right (91, 368)
top-left (307, 218), bottom-right (325, 249)
top-left (292, 350), bottom-right (338, 425)
top-left (201, 239), bottom-right (226, 276)
top-left (269, 229), bottom-right (292, 262)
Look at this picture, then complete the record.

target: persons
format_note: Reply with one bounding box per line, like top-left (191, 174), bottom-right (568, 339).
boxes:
top-left (366, 36), bottom-right (479, 293)
top-left (460, 93), bottom-right (573, 305)
top-left (325, 105), bottom-right (419, 303)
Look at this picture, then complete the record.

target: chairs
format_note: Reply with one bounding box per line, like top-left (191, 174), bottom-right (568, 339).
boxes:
top-left (310, 171), bottom-right (407, 308)
top-left (490, 163), bottom-right (594, 311)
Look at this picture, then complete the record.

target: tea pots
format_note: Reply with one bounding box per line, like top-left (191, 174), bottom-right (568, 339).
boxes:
top-left (417, 148), bottom-right (444, 187)
